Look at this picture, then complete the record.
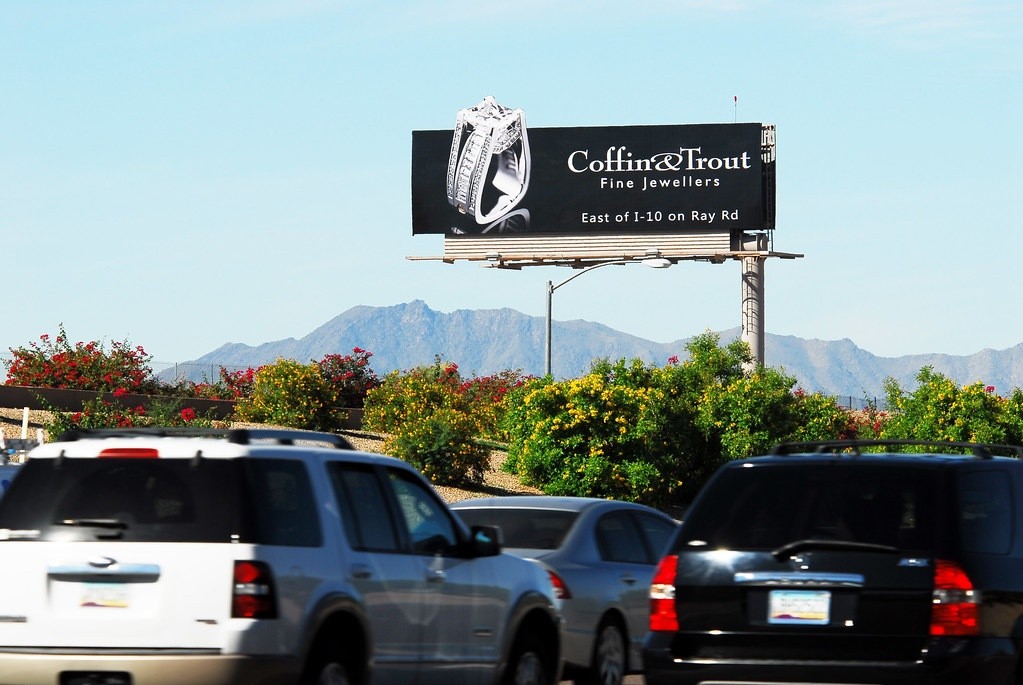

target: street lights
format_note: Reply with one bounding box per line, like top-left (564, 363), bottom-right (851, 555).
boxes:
top-left (543, 257), bottom-right (672, 377)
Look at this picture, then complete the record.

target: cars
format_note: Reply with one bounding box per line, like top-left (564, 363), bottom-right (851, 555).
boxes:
top-left (410, 496), bottom-right (684, 685)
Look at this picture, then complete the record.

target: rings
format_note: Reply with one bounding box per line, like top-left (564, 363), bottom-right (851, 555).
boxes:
top-left (444, 95), bottom-right (531, 224)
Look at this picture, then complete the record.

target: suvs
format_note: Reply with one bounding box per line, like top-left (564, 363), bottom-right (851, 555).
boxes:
top-left (1, 426), bottom-right (562, 685)
top-left (638, 437), bottom-right (1023, 685)
